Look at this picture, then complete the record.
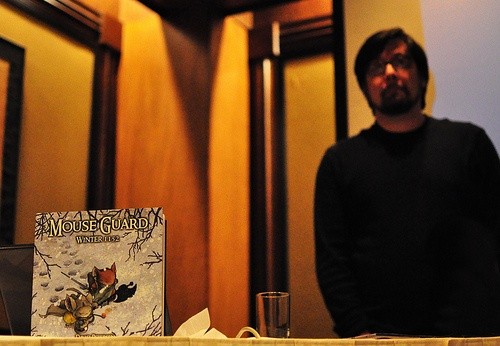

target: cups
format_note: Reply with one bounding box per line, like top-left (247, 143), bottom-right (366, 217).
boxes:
top-left (254, 291), bottom-right (293, 341)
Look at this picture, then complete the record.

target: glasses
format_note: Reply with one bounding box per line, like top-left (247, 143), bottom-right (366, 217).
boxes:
top-left (368, 55), bottom-right (412, 75)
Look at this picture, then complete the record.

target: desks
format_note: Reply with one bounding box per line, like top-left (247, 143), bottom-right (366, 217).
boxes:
top-left (0, 332), bottom-right (498, 346)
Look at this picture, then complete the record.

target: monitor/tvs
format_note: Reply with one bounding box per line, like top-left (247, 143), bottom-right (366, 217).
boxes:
top-left (0, 244), bottom-right (34, 335)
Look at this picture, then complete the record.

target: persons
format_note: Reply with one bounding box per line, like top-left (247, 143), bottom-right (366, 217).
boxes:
top-left (313, 27), bottom-right (500, 338)
